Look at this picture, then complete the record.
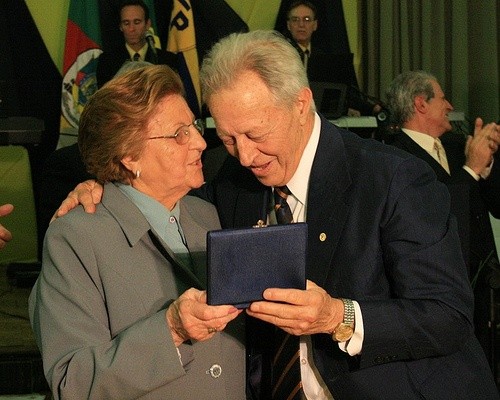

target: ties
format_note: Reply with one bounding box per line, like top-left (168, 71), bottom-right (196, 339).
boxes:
top-left (433, 142), bottom-right (443, 164)
top-left (268, 186), bottom-right (307, 400)
top-left (133, 53), bottom-right (140, 62)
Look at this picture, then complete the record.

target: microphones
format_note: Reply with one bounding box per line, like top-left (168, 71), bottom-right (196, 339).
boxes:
top-left (344, 82), bottom-right (388, 112)
top-left (144, 30), bottom-right (158, 64)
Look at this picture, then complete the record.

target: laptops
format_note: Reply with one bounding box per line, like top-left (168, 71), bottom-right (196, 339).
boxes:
top-left (309, 81), bottom-right (347, 120)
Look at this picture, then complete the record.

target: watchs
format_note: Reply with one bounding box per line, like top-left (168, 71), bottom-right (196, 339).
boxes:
top-left (329, 297), bottom-right (355, 342)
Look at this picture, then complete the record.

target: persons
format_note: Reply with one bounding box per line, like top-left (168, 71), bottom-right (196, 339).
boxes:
top-left (282, 1), bottom-right (389, 122)
top-left (96, 2), bottom-right (181, 89)
top-left (48, 28), bottom-right (500, 400)
top-left (27, 64), bottom-right (249, 400)
top-left (378, 70), bottom-right (500, 393)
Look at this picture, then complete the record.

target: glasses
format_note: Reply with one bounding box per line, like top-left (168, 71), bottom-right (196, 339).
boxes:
top-left (143, 119), bottom-right (205, 145)
top-left (290, 16), bottom-right (312, 25)
